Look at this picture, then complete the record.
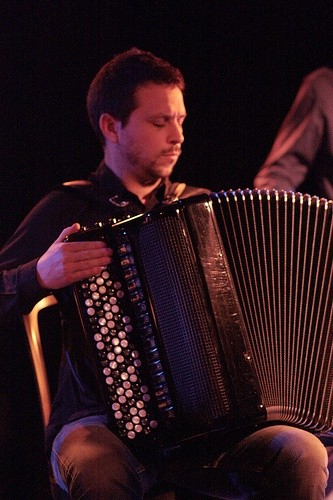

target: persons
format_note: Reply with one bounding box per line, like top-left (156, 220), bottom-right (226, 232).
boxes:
top-left (0, 48), bottom-right (329, 500)
top-left (253, 65), bottom-right (333, 201)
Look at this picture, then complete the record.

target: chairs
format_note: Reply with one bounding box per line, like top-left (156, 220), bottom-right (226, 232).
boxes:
top-left (21, 293), bottom-right (176, 500)
